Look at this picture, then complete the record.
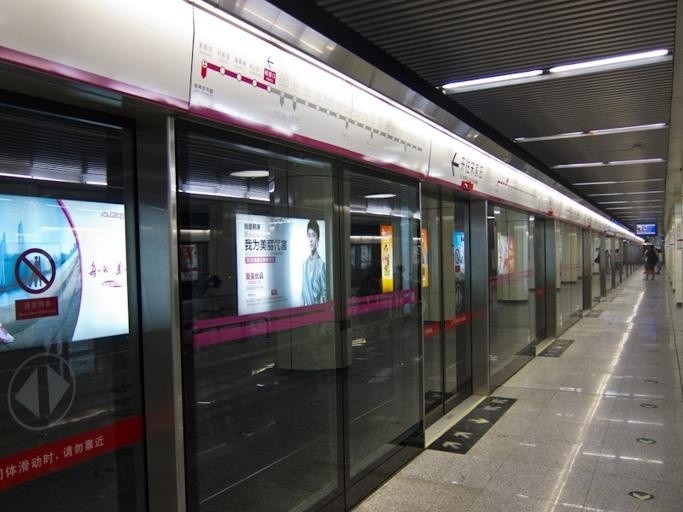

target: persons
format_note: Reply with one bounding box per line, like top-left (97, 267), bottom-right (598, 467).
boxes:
top-left (595, 250), bottom-right (621, 274)
top-left (643, 245), bottom-right (664, 281)
top-left (301, 218), bottom-right (328, 306)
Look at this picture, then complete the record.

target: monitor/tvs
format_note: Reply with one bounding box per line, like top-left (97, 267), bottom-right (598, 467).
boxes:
top-left (635, 223), bottom-right (657, 236)
